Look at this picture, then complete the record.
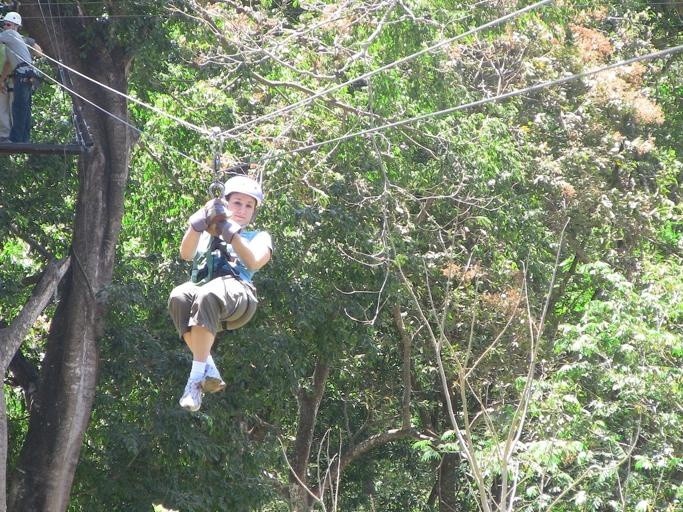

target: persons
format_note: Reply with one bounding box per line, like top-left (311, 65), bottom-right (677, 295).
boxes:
top-left (0, 12), bottom-right (35, 144)
top-left (166, 175), bottom-right (274, 411)
top-left (0, 42), bottom-right (14, 144)
top-left (18, 32), bottom-right (43, 63)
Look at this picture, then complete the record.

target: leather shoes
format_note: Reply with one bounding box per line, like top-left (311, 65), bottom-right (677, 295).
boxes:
top-left (189, 197), bottom-right (242, 244)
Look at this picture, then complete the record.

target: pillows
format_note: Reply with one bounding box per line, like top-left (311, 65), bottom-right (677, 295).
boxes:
top-left (3, 11), bottom-right (21, 25)
top-left (223, 176), bottom-right (263, 208)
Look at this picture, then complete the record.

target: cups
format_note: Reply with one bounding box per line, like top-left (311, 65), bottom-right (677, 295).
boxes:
top-left (3, 23), bottom-right (11, 26)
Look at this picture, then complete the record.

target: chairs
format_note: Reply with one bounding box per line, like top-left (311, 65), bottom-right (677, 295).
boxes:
top-left (179, 365), bottom-right (227, 411)
